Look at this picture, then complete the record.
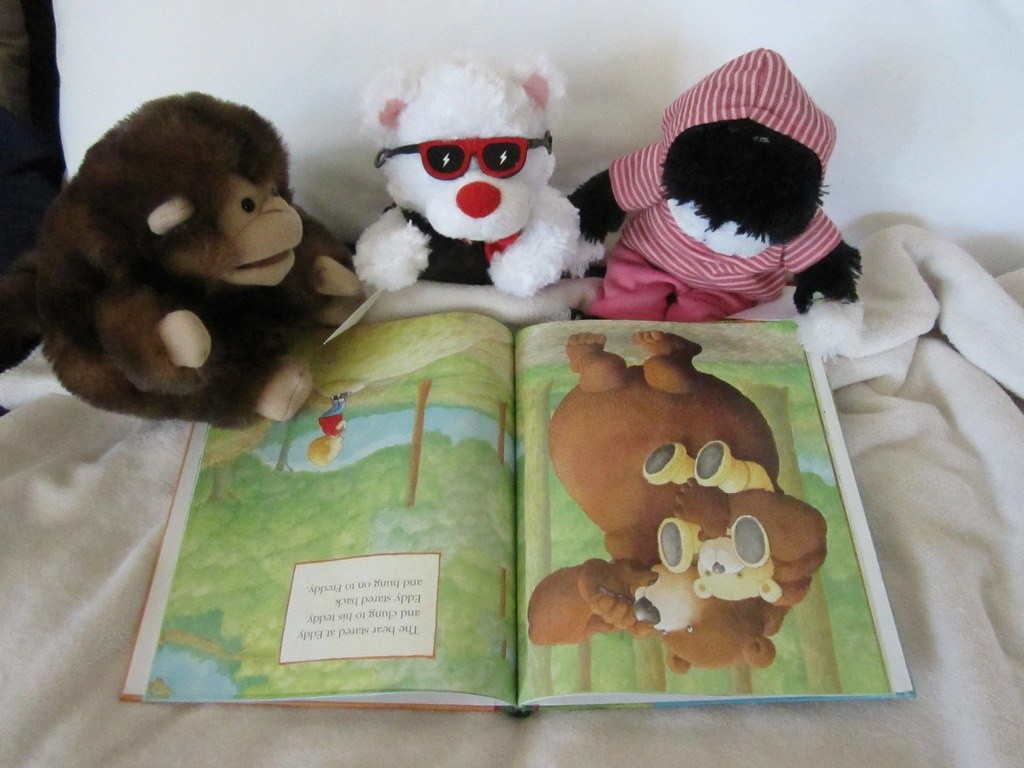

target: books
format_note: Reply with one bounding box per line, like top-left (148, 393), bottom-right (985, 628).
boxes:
top-left (123, 311), bottom-right (917, 709)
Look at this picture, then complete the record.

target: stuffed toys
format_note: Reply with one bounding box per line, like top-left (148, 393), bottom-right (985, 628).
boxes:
top-left (350, 60), bottom-right (604, 295)
top-left (31, 91), bottom-right (358, 430)
top-left (567, 48), bottom-right (861, 363)
